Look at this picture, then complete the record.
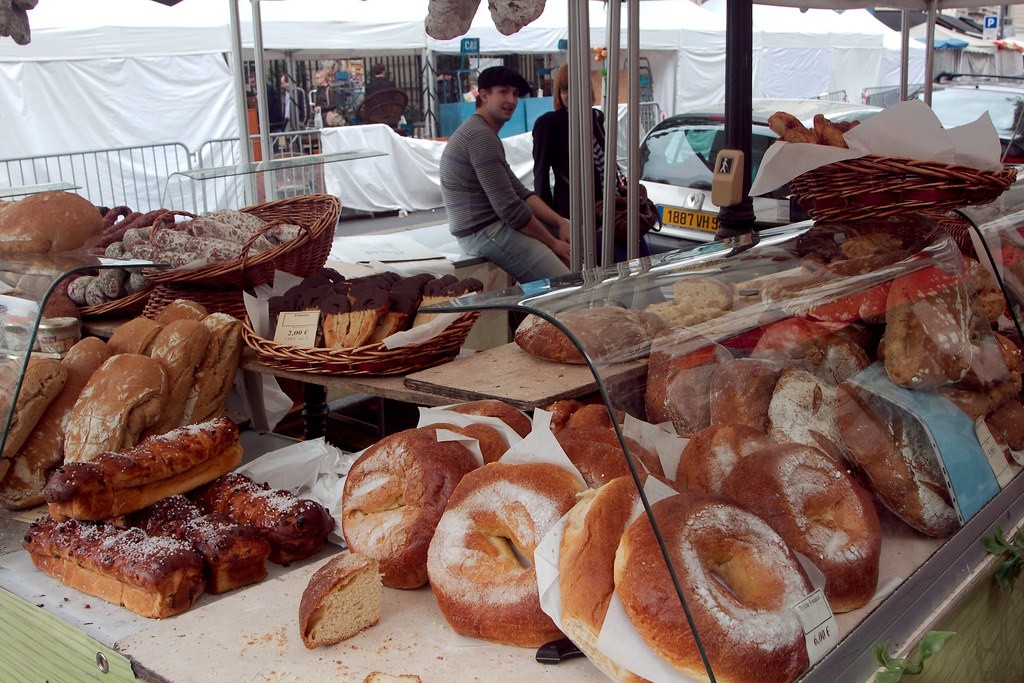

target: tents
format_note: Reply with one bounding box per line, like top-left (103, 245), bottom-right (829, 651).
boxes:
top-left (1, 0), bottom-right (1022, 222)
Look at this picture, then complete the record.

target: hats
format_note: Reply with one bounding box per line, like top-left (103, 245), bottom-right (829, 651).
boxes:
top-left (478, 66), bottom-right (530, 98)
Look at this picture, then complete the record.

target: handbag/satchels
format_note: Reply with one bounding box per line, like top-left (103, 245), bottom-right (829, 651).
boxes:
top-left (595, 183), bottom-right (663, 242)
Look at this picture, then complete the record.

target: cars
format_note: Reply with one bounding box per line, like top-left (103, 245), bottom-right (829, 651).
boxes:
top-left (904, 73), bottom-right (1024, 164)
top-left (637, 100), bottom-right (885, 255)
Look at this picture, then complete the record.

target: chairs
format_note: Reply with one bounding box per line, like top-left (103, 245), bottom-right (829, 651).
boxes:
top-left (270, 153), bottom-right (314, 200)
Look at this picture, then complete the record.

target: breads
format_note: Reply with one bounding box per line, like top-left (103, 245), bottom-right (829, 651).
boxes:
top-left (0, 190), bottom-right (485, 618)
top-left (298, 111), bottom-right (1024, 683)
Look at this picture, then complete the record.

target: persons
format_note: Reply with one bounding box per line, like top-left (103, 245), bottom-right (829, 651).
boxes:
top-left (250, 72), bottom-right (283, 133)
top-left (313, 71), bottom-right (337, 128)
top-left (365, 63), bottom-right (396, 96)
top-left (531, 63), bottom-right (650, 268)
top-left (439, 66), bottom-right (573, 285)
top-left (280, 72), bottom-right (310, 155)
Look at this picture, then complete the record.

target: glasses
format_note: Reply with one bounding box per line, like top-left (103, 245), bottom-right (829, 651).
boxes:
top-left (559, 88), bottom-right (568, 94)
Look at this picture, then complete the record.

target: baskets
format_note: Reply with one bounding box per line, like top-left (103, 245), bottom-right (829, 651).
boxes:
top-left (777, 137), bottom-right (1018, 226)
top-left (139, 193), bottom-right (341, 288)
top-left (142, 282), bottom-right (251, 323)
top-left (241, 309), bottom-right (484, 376)
top-left (76, 287), bottom-right (152, 321)
top-left (918, 211), bottom-right (979, 262)
